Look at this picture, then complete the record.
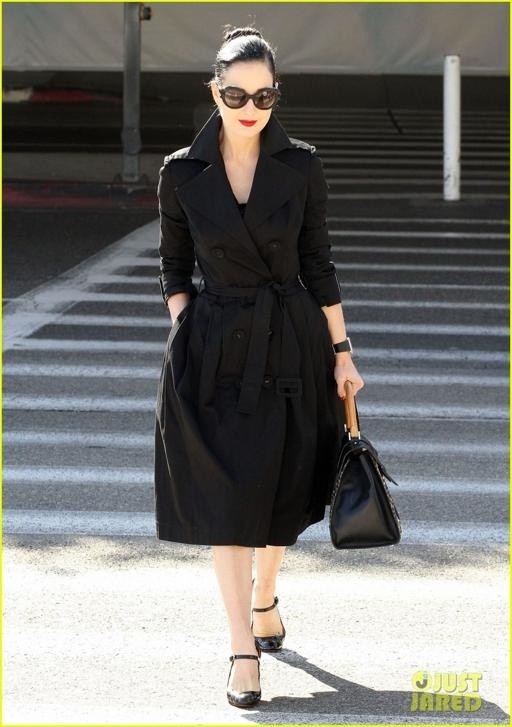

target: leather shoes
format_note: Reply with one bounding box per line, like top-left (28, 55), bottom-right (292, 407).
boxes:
top-left (226, 641), bottom-right (261, 708)
top-left (251, 578), bottom-right (285, 653)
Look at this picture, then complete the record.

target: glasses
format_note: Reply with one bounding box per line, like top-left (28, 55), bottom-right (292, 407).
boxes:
top-left (214, 78), bottom-right (282, 110)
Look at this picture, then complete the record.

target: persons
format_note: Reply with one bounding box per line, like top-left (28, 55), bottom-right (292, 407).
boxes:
top-left (153, 24), bottom-right (365, 709)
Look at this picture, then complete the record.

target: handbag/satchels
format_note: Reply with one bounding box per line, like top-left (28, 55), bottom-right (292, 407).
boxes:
top-left (327, 380), bottom-right (402, 552)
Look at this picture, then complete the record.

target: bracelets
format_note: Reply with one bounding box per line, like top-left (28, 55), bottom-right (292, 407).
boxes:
top-left (332, 336), bottom-right (353, 355)
